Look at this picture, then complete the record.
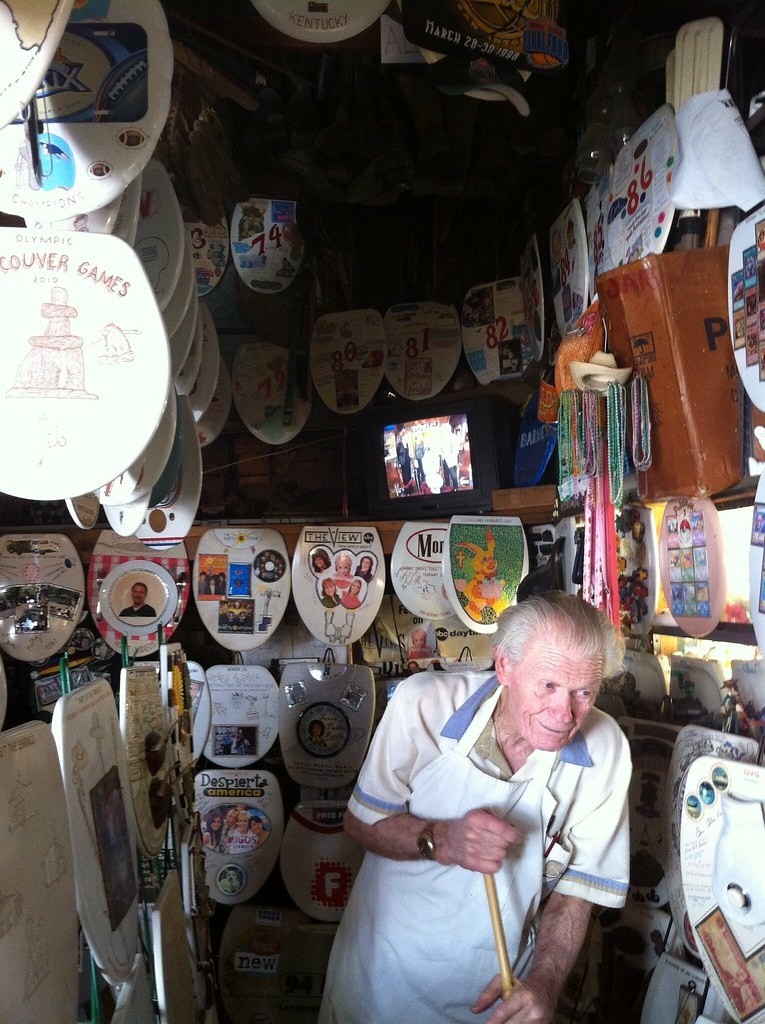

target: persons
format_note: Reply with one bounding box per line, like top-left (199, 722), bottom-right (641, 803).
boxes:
top-left (202, 806), bottom-right (269, 854)
top-left (198, 571), bottom-right (253, 622)
top-left (404, 629), bottom-right (432, 677)
top-left (118, 582), bottom-right (156, 618)
top-left (397, 424), bottom-right (462, 496)
top-left (220, 728), bottom-right (247, 755)
top-left (311, 548), bottom-right (373, 608)
top-left (308, 720), bottom-right (327, 748)
top-left (318, 591), bottom-right (634, 1024)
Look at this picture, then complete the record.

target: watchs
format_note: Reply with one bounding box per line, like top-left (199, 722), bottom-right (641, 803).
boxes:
top-left (418, 821), bottom-right (435, 862)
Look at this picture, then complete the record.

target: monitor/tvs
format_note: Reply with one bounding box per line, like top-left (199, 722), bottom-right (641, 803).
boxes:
top-left (361, 395), bottom-right (520, 521)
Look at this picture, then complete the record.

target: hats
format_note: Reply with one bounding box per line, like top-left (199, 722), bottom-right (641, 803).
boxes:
top-left (537, 366), bottom-right (560, 423)
top-left (554, 300), bottom-right (603, 393)
top-left (569, 351), bottom-right (633, 398)
